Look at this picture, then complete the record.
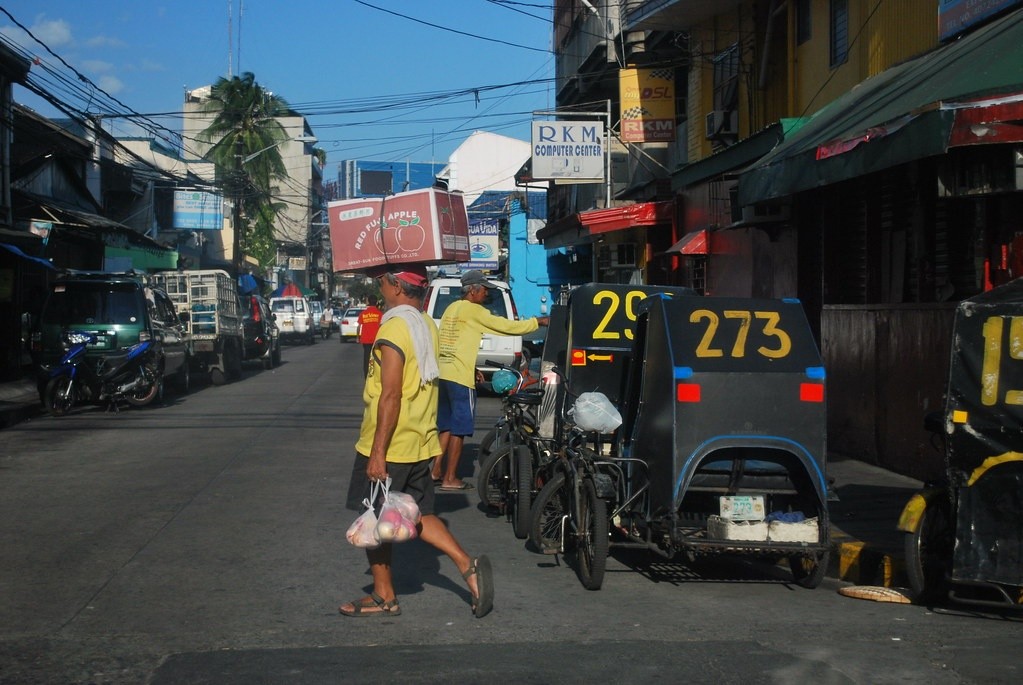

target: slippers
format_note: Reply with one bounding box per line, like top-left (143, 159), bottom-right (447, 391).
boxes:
top-left (439, 480), bottom-right (475, 491)
top-left (432, 476), bottom-right (444, 485)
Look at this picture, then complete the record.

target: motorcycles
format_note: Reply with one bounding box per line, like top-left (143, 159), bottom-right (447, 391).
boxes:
top-left (40, 327), bottom-right (165, 417)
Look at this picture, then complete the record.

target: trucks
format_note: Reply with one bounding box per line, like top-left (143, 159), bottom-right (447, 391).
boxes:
top-left (151, 268), bottom-right (245, 386)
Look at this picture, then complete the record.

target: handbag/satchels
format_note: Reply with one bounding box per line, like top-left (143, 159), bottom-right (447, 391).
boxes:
top-left (566, 391), bottom-right (623, 434)
top-left (345, 475), bottom-right (422, 548)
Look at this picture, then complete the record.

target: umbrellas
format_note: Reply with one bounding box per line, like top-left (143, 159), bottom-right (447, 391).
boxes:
top-left (269, 284), bottom-right (318, 298)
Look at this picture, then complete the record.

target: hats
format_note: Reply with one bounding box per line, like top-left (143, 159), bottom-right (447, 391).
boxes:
top-left (374, 263), bottom-right (429, 287)
top-left (460, 270), bottom-right (497, 289)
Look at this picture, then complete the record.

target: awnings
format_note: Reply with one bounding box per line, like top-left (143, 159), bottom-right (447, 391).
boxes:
top-left (665, 229), bottom-right (709, 255)
top-left (736, 7), bottom-right (1023, 209)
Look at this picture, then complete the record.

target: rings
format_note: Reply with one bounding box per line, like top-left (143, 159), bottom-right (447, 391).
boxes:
top-left (378, 475), bottom-right (381, 477)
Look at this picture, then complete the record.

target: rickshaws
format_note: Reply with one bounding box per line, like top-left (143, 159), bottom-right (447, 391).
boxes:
top-left (478, 278), bottom-right (834, 590)
top-left (892, 275), bottom-right (1022, 616)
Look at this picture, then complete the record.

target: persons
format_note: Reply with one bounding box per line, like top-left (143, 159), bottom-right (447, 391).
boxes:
top-left (357, 295), bottom-right (384, 380)
top-left (338, 265), bottom-right (495, 618)
top-left (431, 269), bottom-right (550, 491)
top-left (322, 305), bottom-right (334, 321)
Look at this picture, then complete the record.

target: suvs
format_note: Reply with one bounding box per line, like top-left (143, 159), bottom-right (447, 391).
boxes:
top-left (24, 271), bottom-right (192, 405)
top-left (423, 274), bottom-right (522, 396)
top-left (238, 293), bottom-right (282, 369)
top-left (266, 295), bottom-right (367, 347)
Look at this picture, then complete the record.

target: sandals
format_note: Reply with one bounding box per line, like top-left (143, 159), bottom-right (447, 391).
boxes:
top-left (338, 593), bottom-right (401, 616)
top-left (461, 553), bottom-right (495, 617)
